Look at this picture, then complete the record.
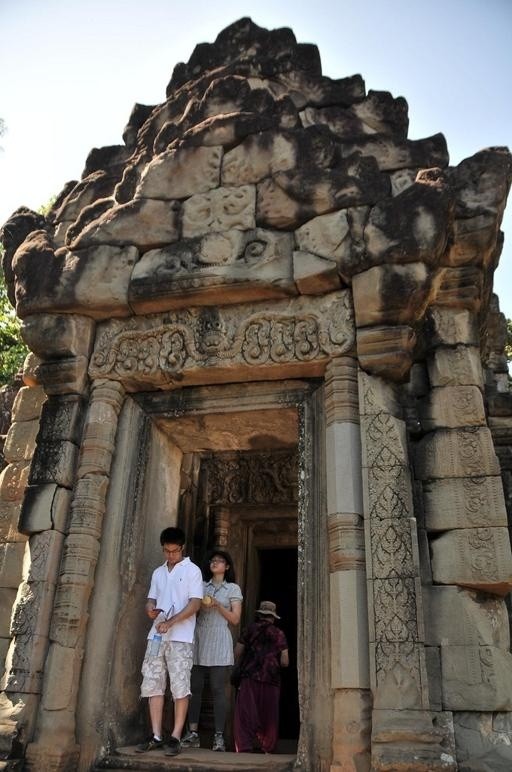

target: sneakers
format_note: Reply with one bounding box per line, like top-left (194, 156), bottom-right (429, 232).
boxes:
top-left (136, 732), bottom-right (227, 757)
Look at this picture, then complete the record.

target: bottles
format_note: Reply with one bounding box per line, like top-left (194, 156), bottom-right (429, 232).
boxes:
top-left (148, 632), bottom-right (162, 657)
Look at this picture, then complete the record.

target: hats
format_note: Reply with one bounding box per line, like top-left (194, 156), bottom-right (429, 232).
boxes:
top-left (255, 600), bottom-right (281, 619)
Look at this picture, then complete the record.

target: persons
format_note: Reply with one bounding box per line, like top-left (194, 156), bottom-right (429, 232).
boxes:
top-left (234, 601), bottom-right (289, 754)
top-left (179, 551), bottom-right (244, 752)
top-left (135, 527), bottom-right (204, 756)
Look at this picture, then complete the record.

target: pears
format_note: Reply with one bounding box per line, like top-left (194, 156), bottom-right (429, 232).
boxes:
top-left (202, 595), bottom-right (212, 605)
